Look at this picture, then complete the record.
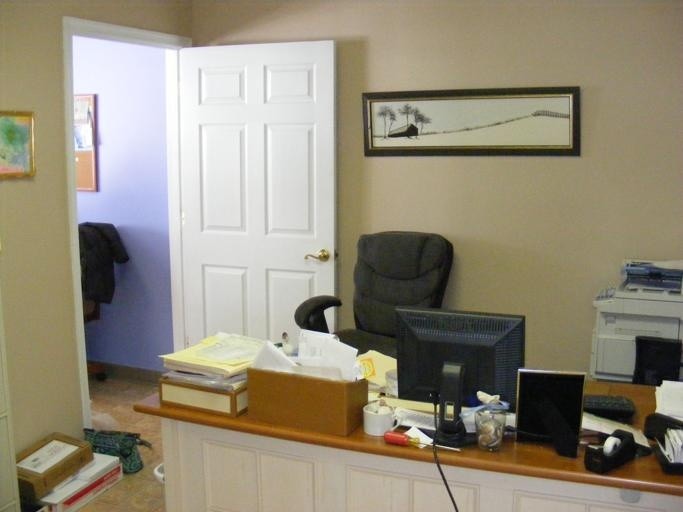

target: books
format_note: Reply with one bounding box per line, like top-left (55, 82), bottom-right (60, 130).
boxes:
top-left (353, 349), bottom-right (398, 393)
top-left (157, 332), bottom-right (265, 395)
top-left (621, 258), bottom-right (683, 292)
top-left (653, 380), bottom-right (683, 422)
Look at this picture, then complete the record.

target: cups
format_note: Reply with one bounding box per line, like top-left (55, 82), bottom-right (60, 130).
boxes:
top-left (363, 402), bottom-right (402, 436)
top-left (474, 406), bottom-right (507, 451)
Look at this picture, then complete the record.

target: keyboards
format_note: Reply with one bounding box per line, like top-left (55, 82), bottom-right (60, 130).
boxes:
top-left (583, 395), bottom-right (636, 419)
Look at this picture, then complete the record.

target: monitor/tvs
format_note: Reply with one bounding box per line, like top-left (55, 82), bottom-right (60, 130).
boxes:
top-left (394, 306), bottom-right (525, 448)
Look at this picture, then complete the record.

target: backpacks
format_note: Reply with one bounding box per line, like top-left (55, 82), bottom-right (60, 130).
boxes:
top-left (83, 428), bottom-right (151, 475)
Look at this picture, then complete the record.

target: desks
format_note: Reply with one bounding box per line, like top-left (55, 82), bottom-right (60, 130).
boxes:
top-left (131, 381), bottom-right (683, 512)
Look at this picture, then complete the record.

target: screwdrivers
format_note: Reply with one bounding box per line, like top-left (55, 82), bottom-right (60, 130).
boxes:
top-left (384, 431), bottom-right (461, 452)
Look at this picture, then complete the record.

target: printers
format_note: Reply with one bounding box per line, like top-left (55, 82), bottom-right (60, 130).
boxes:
top-left (589, 263), bottom-right (682, 384)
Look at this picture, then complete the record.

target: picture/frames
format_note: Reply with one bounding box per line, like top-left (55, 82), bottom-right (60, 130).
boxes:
top-left (362, 85), bottom-right (582, 157)
top-left (0, 109), bottom-right (37, 183)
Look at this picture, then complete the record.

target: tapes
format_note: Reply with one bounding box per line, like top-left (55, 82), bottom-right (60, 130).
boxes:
top-left (604, 437), bottom-right (621, 454)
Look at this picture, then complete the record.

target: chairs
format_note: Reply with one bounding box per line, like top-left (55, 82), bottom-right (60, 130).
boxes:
top-left (294, 231), bottom-right (454, 359)
top-left (79, 222), bottom-right (129, 384)
